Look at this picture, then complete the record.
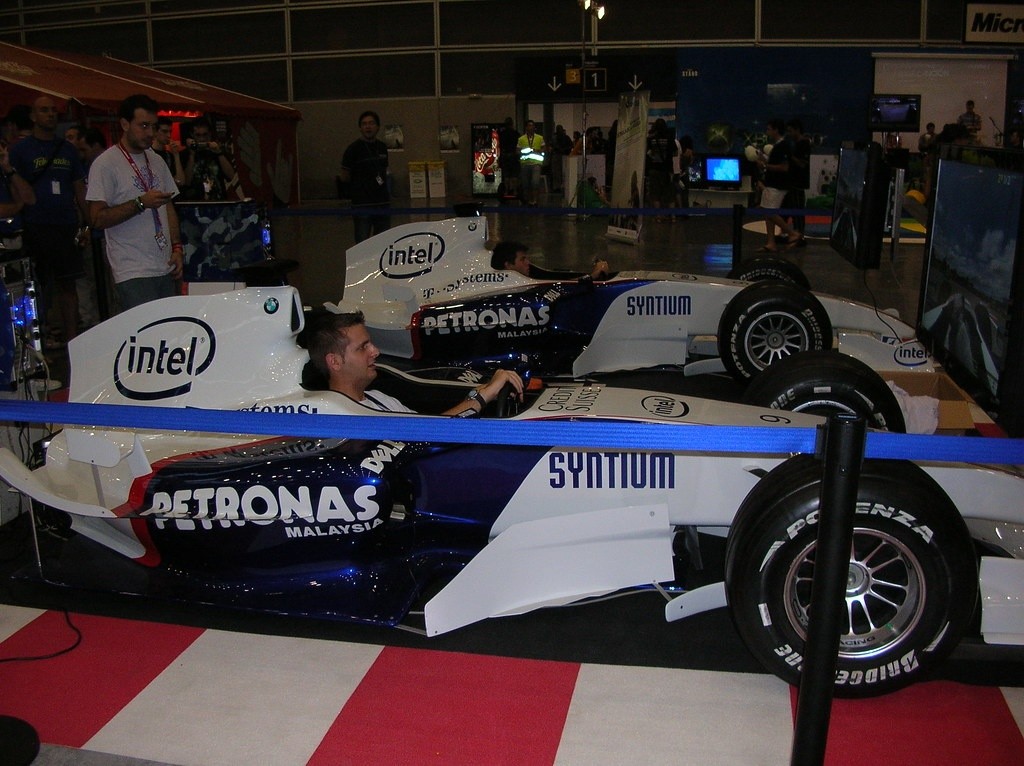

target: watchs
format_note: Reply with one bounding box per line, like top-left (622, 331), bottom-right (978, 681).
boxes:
top-left (469, 390), bottom-right (487, 408)
top-left (7, 168), bottom-right (17, 176)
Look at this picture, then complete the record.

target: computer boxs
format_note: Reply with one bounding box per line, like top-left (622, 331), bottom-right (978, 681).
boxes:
top-left (0, 280), bottom-right (45, 382)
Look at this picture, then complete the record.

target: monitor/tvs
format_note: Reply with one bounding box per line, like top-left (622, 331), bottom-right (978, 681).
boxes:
top-left (869, 94), bottom-right (921, 133)
top-left (706, 158), bottom-right (742, 191)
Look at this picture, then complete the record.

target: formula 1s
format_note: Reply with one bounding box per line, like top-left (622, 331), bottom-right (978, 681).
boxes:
top-left (321, 216), bottom-right (939, 394)
top-left (0, 285), bottom-right (1023, 699)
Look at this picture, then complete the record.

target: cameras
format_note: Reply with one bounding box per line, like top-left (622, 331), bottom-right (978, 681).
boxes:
top-left (191, 140), bottom-right (209, 151)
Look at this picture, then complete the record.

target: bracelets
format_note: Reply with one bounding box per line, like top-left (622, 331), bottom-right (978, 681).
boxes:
top-left (172, 243), bottom-right (182, 248)
top-left (135, 197), bottom-right (146, 214)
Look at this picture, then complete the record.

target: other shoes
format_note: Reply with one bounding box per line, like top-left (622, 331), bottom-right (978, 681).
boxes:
top-left (652, 216), bottom-right (663, 224)
top-left (671, 218), bottom-right (677, 222)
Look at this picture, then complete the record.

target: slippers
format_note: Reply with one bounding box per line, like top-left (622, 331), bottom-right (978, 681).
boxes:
top-left (756, 246), bottom-right (778, 253)
top-left (784, 236), bottom-right (803, 250)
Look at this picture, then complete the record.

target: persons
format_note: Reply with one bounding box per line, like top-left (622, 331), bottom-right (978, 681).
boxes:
top-left (500, 117), bottom-right (618, 206)
top-left (649, 119), bottom-right (694, 224)
top-left (86, 95), bottom-right (185, 304)
top-left (0, 97), bottom-right (108, 341)
top-left (1009, 129), bottom-right (1024, 148)
top-left (308, 311), bottom-right (524, 416)
top-left (149, 117), bottom-right (234, 281)
top-left (918, 123), bottom-right (995, 170)
top-left (340, 111), bottom-right (391, 236)
top-left (958, 100), bottom-right (981, 133)
top-left (752, 119), bottom-right (810, 254)
top-left (492, 241), bottom-right (608, 280)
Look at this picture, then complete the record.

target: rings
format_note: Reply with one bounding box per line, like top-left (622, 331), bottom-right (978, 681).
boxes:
top-left (602, 265), bottom-right (604, 268)
